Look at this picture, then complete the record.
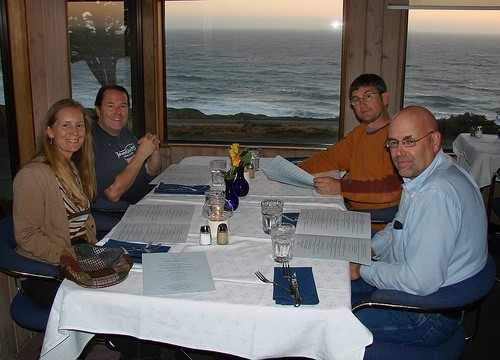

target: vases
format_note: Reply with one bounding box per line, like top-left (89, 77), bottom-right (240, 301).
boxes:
top-left (233, 166), bottom-right (249, 197)
top-left (224, 179), bottom-right (239, 211)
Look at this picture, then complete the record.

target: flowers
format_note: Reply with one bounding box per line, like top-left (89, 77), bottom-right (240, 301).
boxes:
top-left (219, 142), bottom-right (252, 180)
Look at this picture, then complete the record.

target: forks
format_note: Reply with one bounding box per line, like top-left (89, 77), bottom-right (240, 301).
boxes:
top-left (255, 270), bottom-right (290, 292)
top-left (283, 261), bottom-right (296, 298)
top-left (126, 243), bottom-right (161, 252)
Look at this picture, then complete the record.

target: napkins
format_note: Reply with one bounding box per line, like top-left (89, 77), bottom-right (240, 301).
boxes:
top-left (103, 239), bottom-right (171, 264)
top-left (273, 267), bottom-right (319, 305)
top-left (282, 213), bottom-right (300, 227)
top-left (154, 181), bottom-right (210, 195)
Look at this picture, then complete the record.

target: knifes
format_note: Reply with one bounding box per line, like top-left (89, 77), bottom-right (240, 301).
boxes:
top-left (291, 271), bottom-right (302, 307)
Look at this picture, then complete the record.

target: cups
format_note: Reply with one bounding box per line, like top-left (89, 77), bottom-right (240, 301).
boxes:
top-left (271, 223), bottom-right (295, 263)
top-left (248, 148), bottom-right (261, 171)
top-left (210, 160), bottom-right (227, 186)
top-left (261, 198), bottom-right (284, 234)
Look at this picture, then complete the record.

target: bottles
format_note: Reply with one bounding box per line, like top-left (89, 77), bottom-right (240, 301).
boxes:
top-left (217, 223), bottom-right (229, 245)
top-left (200, 226), bottom-right (212, 246)
top-left (470, 124), bottom-right (482, 138)
top-left (248, 164), bottom-right (255, 179)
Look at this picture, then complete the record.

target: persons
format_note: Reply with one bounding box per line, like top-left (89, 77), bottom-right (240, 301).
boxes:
top-left (294, 74), bottom-right (405, 237)
top-left (85, 85), bottom-right (161, 230)
top-left (350, 105), bottom-right (488, 348)
top-left (12, 98), bottom-right (122, 351)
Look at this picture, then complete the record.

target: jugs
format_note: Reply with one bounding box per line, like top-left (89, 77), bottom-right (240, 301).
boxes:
top-left (203, 190), bottom-right (234, 221)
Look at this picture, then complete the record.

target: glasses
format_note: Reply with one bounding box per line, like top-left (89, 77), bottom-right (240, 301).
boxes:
top-left (350, 91), bottom-right (383, 105)
top-left (385, 131), bottom-right (434, 148)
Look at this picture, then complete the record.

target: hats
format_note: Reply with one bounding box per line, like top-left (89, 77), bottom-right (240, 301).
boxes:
top-left (60, 243), bottom-right (133, 288)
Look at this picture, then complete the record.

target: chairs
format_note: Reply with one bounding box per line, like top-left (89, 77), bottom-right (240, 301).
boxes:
top-left (91, 197), bottom-right (130, 233)
top-left (0, 214), bottom-right (106, 360)
top-left (352, 253), bottom-right (495, 360)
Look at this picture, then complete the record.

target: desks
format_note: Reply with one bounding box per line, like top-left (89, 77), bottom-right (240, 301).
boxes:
top-left (452, 132), bottom-right (500, 238)
top-left (38, 156), bottom-right (373, 360)
